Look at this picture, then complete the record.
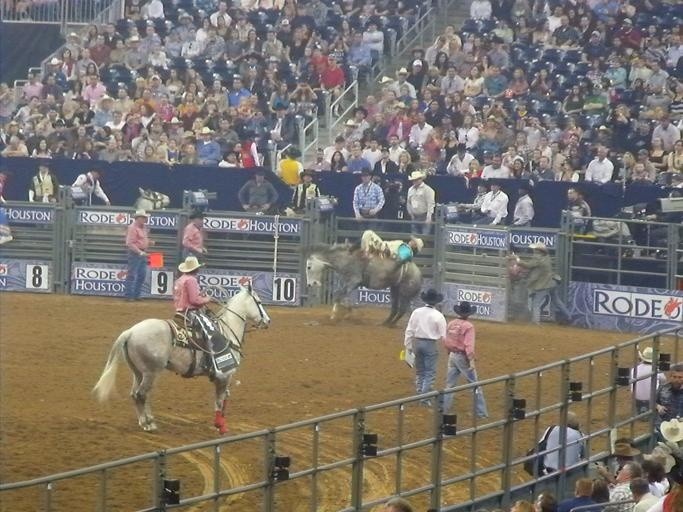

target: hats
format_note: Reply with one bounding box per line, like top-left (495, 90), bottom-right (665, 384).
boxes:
top-left (189, 209), bottom-right (207, 219)
top-left (410, 235), bottom-right (424, 253)
top-left (299, 168), bottom-right (315, 178)
top-left (659, 419), bottom-right (682, 442)
top-left (613, 442), bottom-right (680, 474)
top-left (421, 288), bottom-right (444, 305)
top-left (130, 209), bottom-right (151, 218)
top-left (178, 256), bottom-right (202, 273)
top-left (528, 241), bottom-right (548, 254)
top-left (453, 301), bottom-right (477, 318)
top-left (638, 347), bottom-right (661, 363)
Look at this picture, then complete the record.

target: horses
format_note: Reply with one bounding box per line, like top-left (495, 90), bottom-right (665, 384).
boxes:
top-left (295, 239), bottom-right (424, 328)
top-left (85, 186), bottom-right (170, 237)
top-left (90, 279), bottom-right (271, 435)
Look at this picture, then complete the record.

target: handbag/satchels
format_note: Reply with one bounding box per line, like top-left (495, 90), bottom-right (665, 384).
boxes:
top-left (524, 425), bottom-right (556, 478)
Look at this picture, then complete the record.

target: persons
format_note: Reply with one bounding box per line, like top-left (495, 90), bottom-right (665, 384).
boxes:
top-left (506, 242), bottom-right (572, 329)
top-left (176, 212), bottom-right (208, 285)
top-left (1, 167), bottom-right (16, 203)
top-left (360, 229), bottom-right (424, 277)
top-left (28, 161), bottom-right (111, 206)
top-left (352, 169), bottom-right (435, 236)
top-left (566, 187), bottom-right (592, 234)
top-left (403, 289), bottom-right (448, 407)
top-left (384, 497), bottom-right (413, 512)
top-left (171, 257), bottom-right (226, 383)
top-left (510, 347), bottom-right (683, 511)
top-left (458, 180), bottom-right (535, 228)
top-left (2, 0), bottom-right (432, 167)
top-left (320, 0), bottom-right (683, 179)
top-left (444, 302), bottom-right (493, 421)
top-left (238, 146), bottom-right (320, 216)
top-left (124, 210), bottom-right (155, 303)
